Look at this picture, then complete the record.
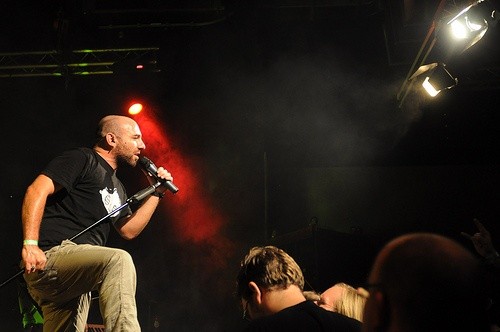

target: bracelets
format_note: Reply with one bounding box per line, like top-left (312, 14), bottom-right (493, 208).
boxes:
top-left (23, 239), bottom-right (39, 246)
top-left (150, 191), bottom-right (166, 199)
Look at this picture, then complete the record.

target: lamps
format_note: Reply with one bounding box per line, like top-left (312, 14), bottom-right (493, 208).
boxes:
top-left (445, 0), bottom-right (500, 52)
top-left (408, 55), bottom-right (465, 98)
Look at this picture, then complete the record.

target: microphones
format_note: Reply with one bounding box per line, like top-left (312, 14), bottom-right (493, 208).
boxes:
top-left (136, 155), bottom-right (179, 194)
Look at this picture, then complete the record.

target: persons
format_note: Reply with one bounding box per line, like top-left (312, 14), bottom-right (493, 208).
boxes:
top-left (18, 115), bottom-right (174, 332)
top-left (360, 233), bottom-right (492, 332)
top-left (313, 282), bottom-right (367, 322)
top-left (464, 219), bottom-right (499, 266)
top-left (233, 246), bottom-right (363, 332)
